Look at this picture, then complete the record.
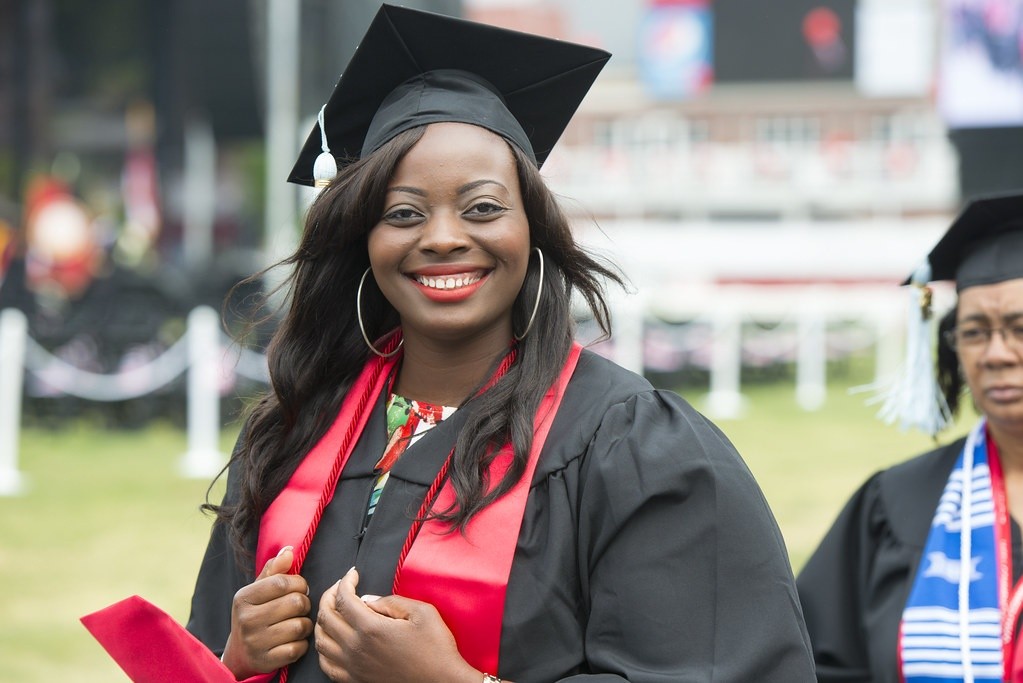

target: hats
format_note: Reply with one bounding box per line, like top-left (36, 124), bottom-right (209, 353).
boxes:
top-left (284, 2), bottom-right (610, 201)
top-left (876, 123), bottom-right (1023, 431)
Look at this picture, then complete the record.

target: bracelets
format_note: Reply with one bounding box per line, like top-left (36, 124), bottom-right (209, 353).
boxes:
top-left (483, 673), bottom-right (501, 683)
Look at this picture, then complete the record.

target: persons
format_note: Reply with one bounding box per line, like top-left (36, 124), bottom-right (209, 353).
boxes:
top-left (795, 191), bottom-right (1023, 683)
top-left (76, 4), bottom-right (820, 683)
